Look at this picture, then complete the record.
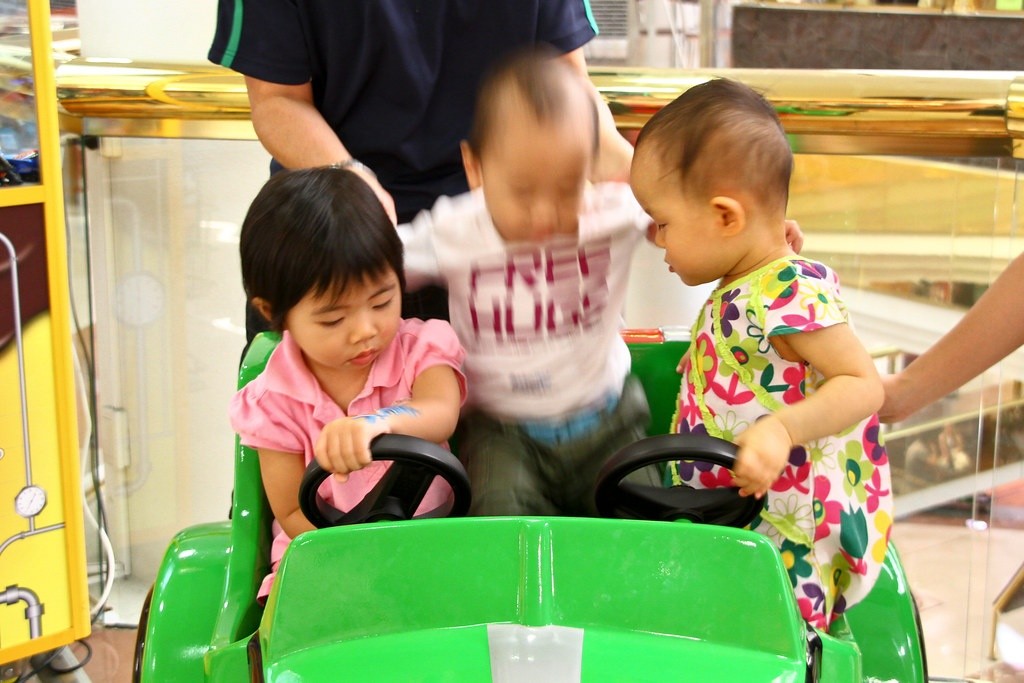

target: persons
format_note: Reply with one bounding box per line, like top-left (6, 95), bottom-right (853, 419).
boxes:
top-left (878, 251), bottom-right (1024, 425)
top-left (221, 167), bottom-right (470, 607)
top-left (628, 77), bottom-right (895, 666)
top-left (390, 42), bottom-right (805, 520)
top-left (209, 0), bottom-right (634, 340)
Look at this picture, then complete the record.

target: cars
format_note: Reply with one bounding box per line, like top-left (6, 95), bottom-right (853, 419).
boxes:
top-left (134, 306), bottom-right (935, 682)
